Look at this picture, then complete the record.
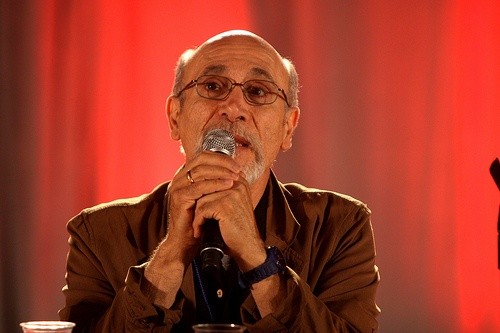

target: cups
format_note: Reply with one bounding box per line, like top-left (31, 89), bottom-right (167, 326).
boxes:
top-left (192, 324), bottom-right (246, 333)
top-left (19, 320), bottom-right (76, 333)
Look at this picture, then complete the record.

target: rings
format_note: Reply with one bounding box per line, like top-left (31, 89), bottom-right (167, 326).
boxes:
top-left (187, 169), bottom-right (195, 184)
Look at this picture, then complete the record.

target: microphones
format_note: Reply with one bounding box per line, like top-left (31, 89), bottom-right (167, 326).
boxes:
top-left (201, 129), bottom-right (235, 260)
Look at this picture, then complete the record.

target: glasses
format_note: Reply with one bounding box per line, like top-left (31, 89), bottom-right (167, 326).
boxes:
top-left (176, 74), bottom-right (291, 108)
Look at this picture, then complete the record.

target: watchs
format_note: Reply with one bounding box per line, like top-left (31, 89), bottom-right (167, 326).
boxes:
top-left (239, 245), bottom-right (287, 288)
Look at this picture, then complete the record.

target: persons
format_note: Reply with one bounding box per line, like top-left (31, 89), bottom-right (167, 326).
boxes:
top-left (58, 30), bottom-right (381, 333)
